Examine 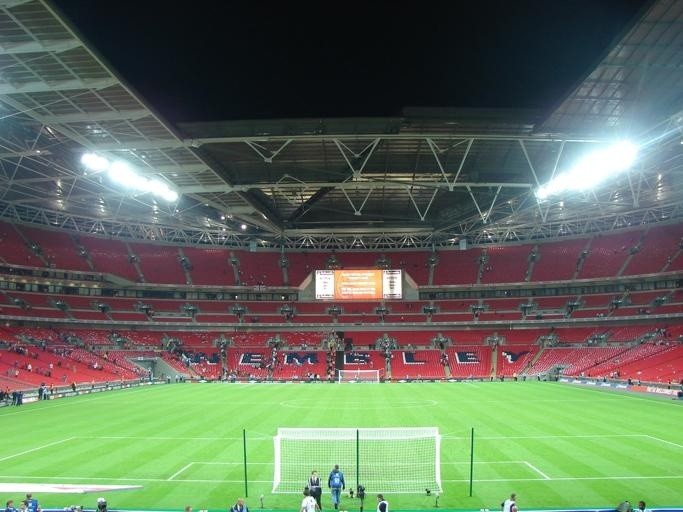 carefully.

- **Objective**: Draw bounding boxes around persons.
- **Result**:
[95,498,107,512]
[4,493,41,512]
[635,501,652,512]
[230,498,249,512]
[0,330,682,407]
[329,465,345,509]
[307,471,322,510]
[300,490,320,512]
[377,494,388,512]
[185,505,193,512]
[501,493,517,512]
